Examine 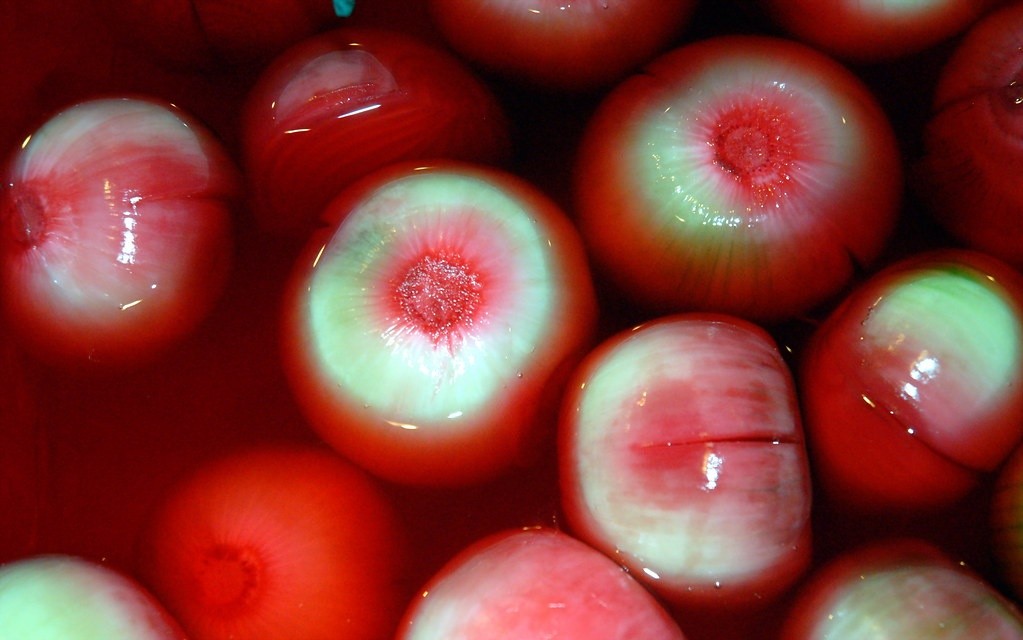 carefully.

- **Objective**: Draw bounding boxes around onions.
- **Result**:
[0,0,1023,640]
